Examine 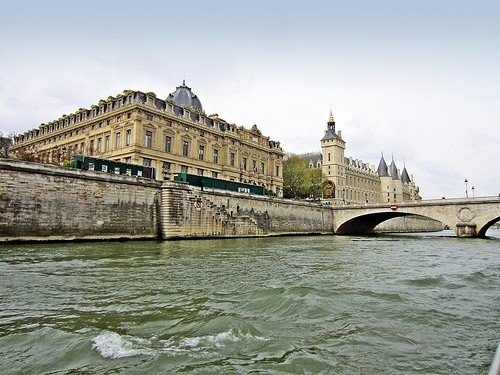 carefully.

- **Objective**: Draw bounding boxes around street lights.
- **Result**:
[472,186,475,197]
[464,179,468,198]
